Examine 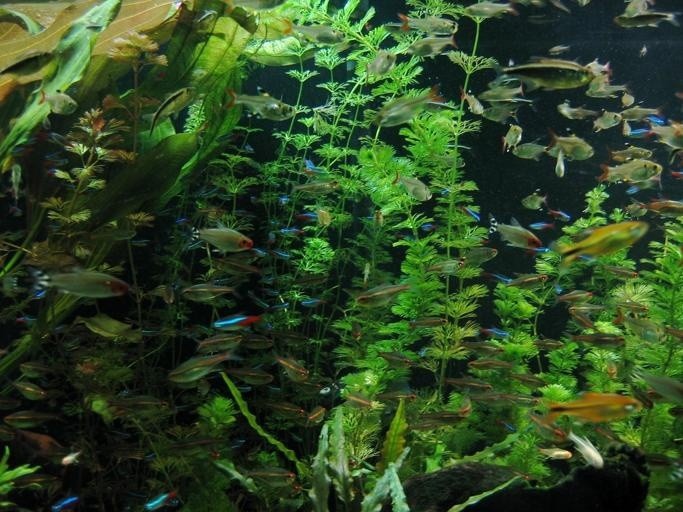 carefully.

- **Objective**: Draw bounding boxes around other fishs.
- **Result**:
[0,0,683,511]
